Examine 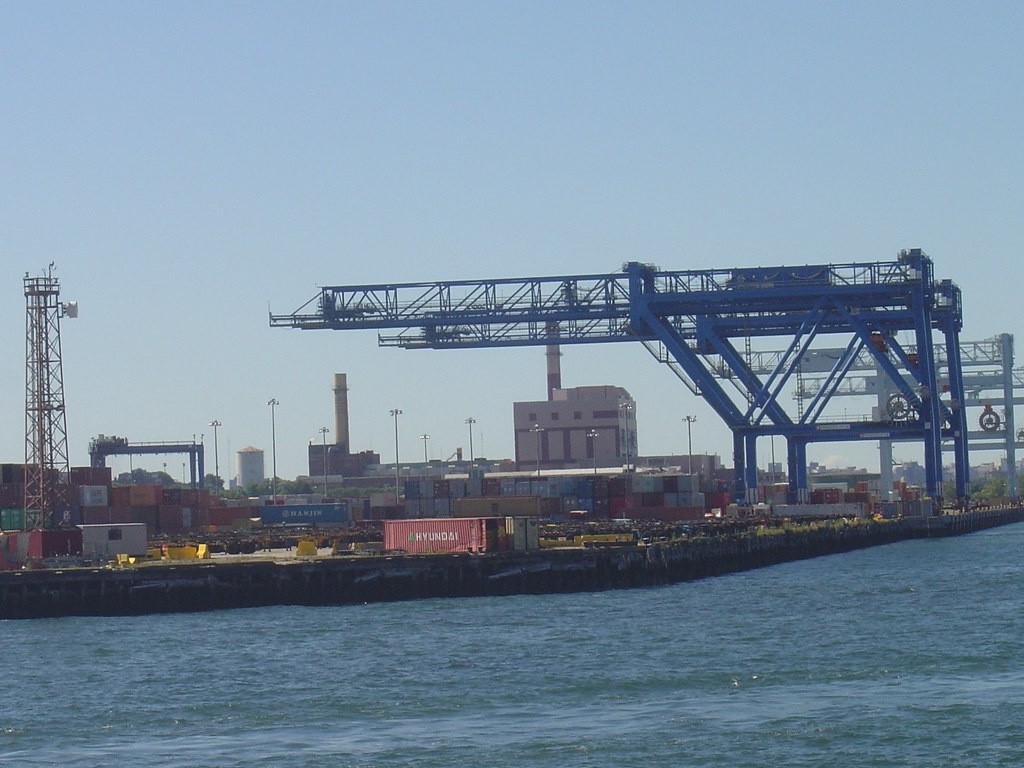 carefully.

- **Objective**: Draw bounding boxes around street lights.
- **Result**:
[266,398,280,505]
[419,433,430,463]
[586,429,599,476]
[389,408,403,504]
[208,419,222,495]
[464,417,476,468]
[318,427,330,496]
[530,424,544,476]
[182,463,185,486]
[620,402,633,474]
[682,413,698,474]
